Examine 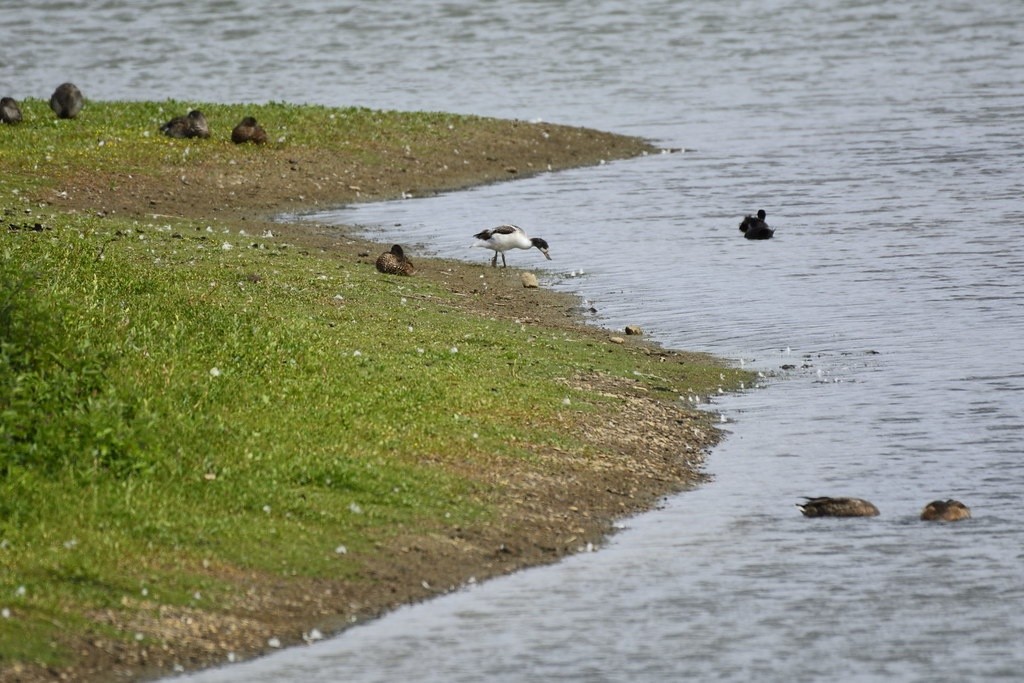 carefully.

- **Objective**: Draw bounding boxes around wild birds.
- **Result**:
[50,83,85,120]
[376,244,421,277]
[0,97,24,126]
[920,497,970,523]
[794,495,881,519]
[159,110,211,140]
[468,224,552,269]
[231,117,269,146]
[739,209,777,240]
